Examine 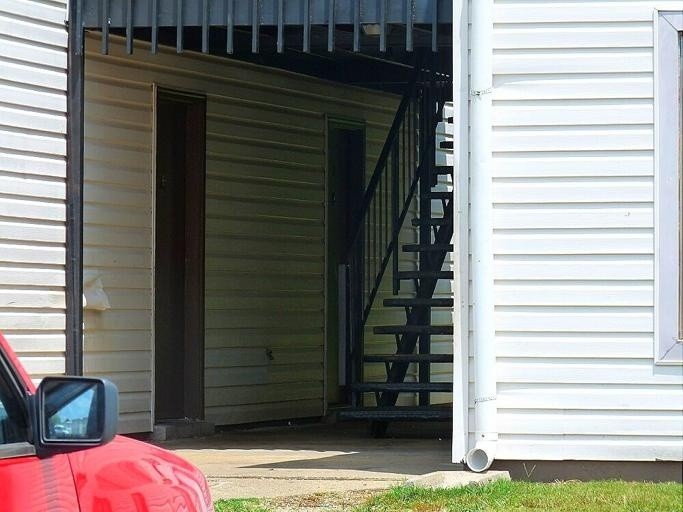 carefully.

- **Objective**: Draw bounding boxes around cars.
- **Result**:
[0,328,214,512]
